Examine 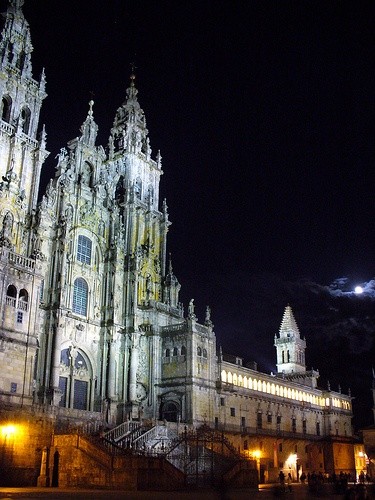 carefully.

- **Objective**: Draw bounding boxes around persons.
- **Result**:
[278,469,375,500]
